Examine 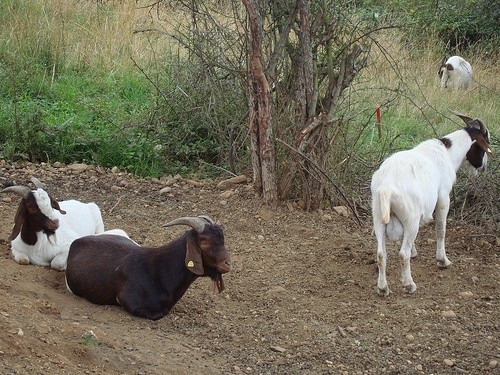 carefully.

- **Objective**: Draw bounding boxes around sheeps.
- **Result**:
[437,55,472,99]
[1,177,104,272]
[64,214,231,321]
[370,113,493,298]
